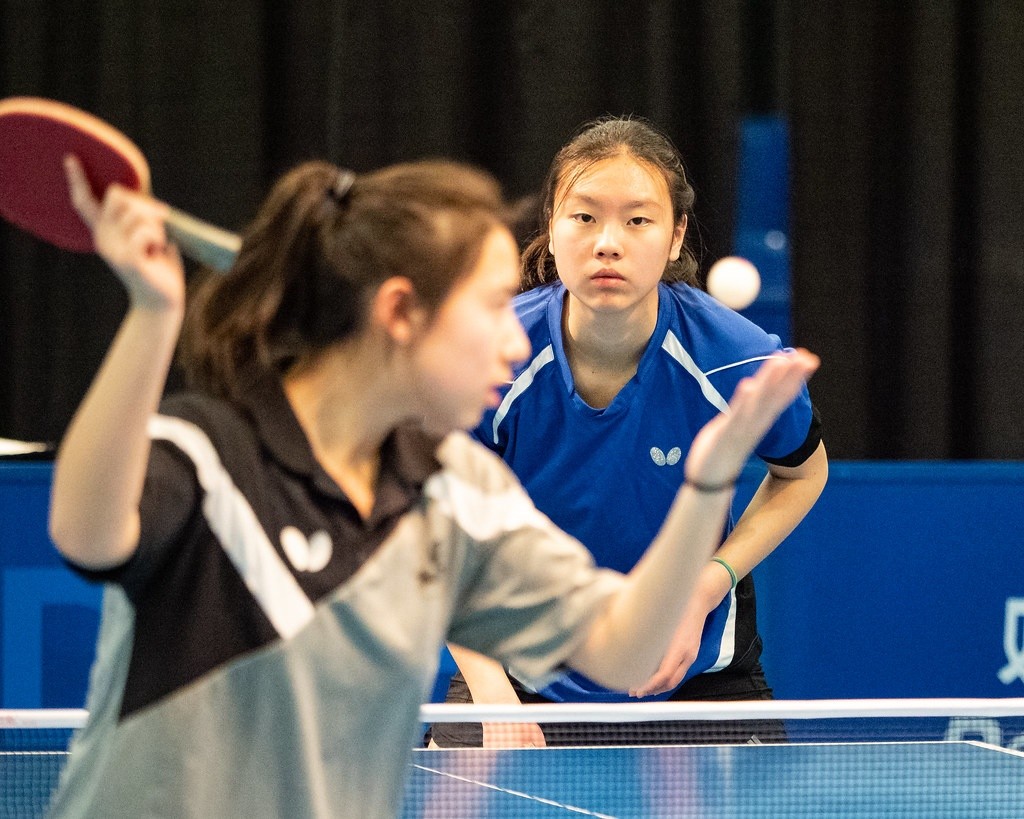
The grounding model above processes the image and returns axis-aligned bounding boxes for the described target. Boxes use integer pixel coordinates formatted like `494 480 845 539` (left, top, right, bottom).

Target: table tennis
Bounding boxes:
707 253 763 304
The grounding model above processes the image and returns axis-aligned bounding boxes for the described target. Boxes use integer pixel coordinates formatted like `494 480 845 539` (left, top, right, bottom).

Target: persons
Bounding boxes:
423 115 829 750
43 154 818 819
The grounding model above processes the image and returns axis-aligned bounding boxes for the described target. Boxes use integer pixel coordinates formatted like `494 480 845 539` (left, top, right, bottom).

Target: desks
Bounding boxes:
0 740 1024 818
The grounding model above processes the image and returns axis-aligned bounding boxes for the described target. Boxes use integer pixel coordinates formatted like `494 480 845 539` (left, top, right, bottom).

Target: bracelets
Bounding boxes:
680 469 741 498
710 555 736 591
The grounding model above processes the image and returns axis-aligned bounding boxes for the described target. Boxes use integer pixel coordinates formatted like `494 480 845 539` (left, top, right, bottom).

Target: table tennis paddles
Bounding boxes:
0 96 245 274
